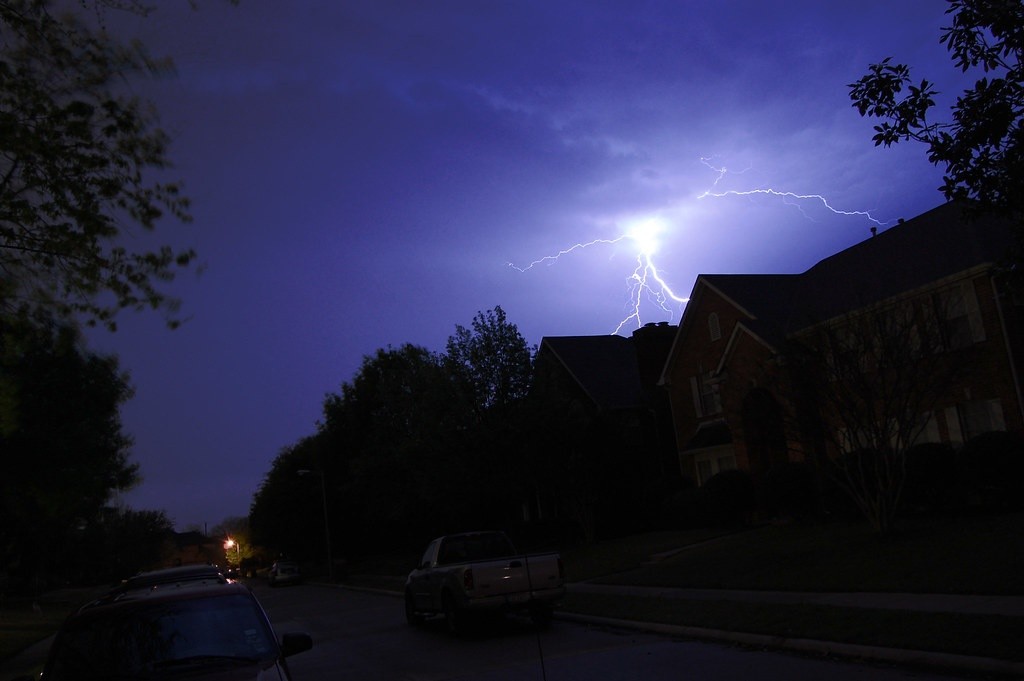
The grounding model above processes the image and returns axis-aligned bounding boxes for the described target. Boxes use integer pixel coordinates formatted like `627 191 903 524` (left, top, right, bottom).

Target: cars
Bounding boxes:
11 573 312 681
96 545 307 605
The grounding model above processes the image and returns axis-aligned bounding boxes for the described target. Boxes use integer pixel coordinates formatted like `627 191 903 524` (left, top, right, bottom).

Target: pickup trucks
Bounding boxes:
404 531 570 638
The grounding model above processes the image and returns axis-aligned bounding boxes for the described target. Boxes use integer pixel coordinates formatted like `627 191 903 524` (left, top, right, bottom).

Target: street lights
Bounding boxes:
297 470 333 582
